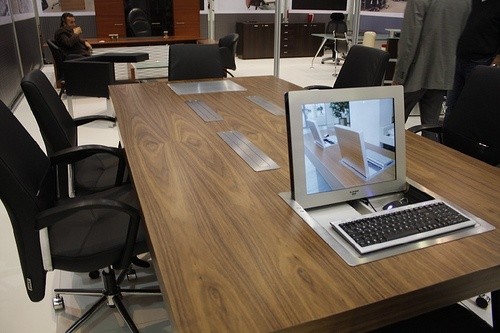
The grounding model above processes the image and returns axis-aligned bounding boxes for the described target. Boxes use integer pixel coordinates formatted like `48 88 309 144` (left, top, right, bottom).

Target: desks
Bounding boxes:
310 33 413 72
304 128 396 189
94 50 149 79
107 74 500 333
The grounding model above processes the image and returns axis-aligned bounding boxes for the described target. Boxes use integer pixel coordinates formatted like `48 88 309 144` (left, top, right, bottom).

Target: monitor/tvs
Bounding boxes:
285 84 407 209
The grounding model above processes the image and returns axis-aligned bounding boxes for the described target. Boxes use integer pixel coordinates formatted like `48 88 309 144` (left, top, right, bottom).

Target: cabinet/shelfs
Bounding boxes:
235 21 326 60
94 0 199 37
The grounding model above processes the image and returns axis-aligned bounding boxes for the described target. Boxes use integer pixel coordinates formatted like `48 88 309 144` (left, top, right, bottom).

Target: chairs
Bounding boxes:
408 66 500 167
167 46 237 79
321 12 349 64
47 39 89 88
128 8 154 37
219 34 240 78
304 45 391 91
0 69 153 333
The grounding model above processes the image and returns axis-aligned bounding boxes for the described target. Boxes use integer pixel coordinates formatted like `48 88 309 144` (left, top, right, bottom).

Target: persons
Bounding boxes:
55 12 93 56
392 0 471 140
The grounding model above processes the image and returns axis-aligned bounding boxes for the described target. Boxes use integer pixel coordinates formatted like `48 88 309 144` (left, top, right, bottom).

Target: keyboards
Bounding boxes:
330 199 476 254
